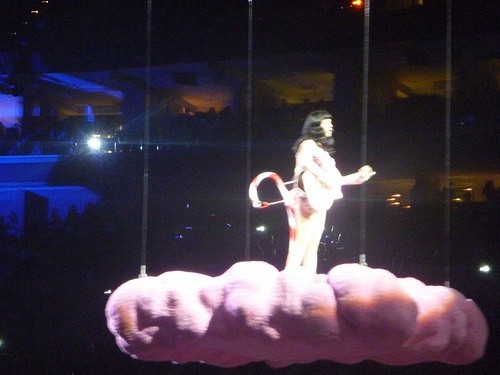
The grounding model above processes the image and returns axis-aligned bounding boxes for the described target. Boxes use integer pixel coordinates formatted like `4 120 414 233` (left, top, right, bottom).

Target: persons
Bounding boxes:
279 108 376 273
